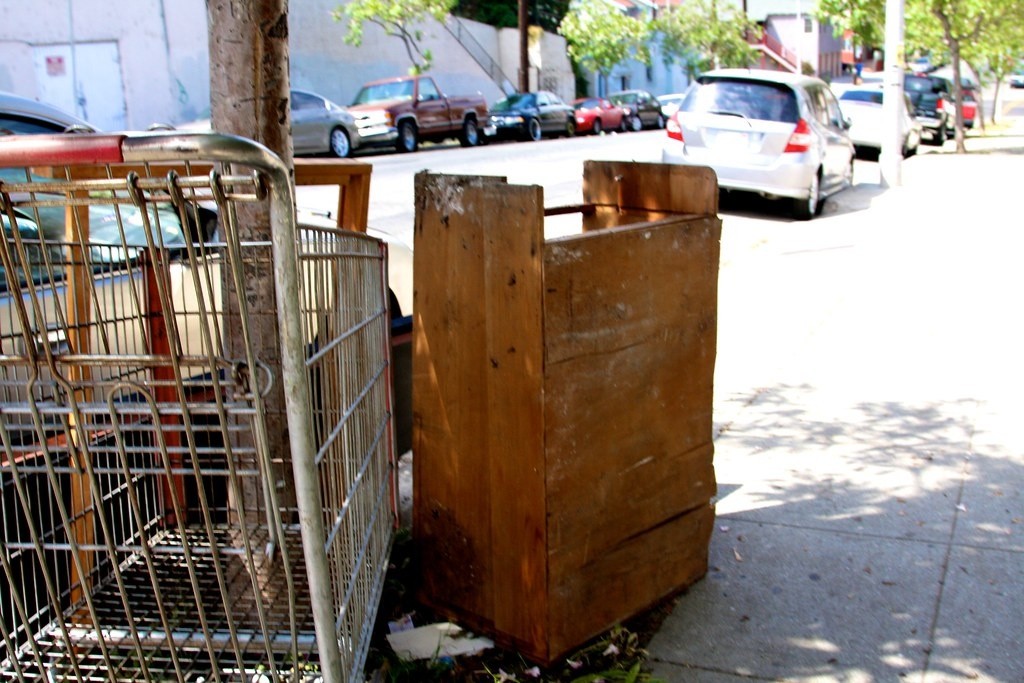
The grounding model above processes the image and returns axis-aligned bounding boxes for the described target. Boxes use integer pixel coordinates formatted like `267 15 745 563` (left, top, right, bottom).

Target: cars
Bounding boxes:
476 91 574 145
661 68 857 221
839 73 977 156
1 92 413 436
569 97 628 136
179 89 361 161
608 90 687 132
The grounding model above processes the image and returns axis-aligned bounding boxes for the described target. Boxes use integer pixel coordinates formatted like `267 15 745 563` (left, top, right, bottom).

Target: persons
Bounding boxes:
854 58 865 84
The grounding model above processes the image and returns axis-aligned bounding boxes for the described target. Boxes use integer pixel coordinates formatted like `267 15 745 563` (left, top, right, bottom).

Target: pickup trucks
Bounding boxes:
343 74 490 152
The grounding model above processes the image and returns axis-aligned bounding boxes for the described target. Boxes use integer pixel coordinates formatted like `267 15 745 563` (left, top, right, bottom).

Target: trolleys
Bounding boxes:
0 125 402 683
